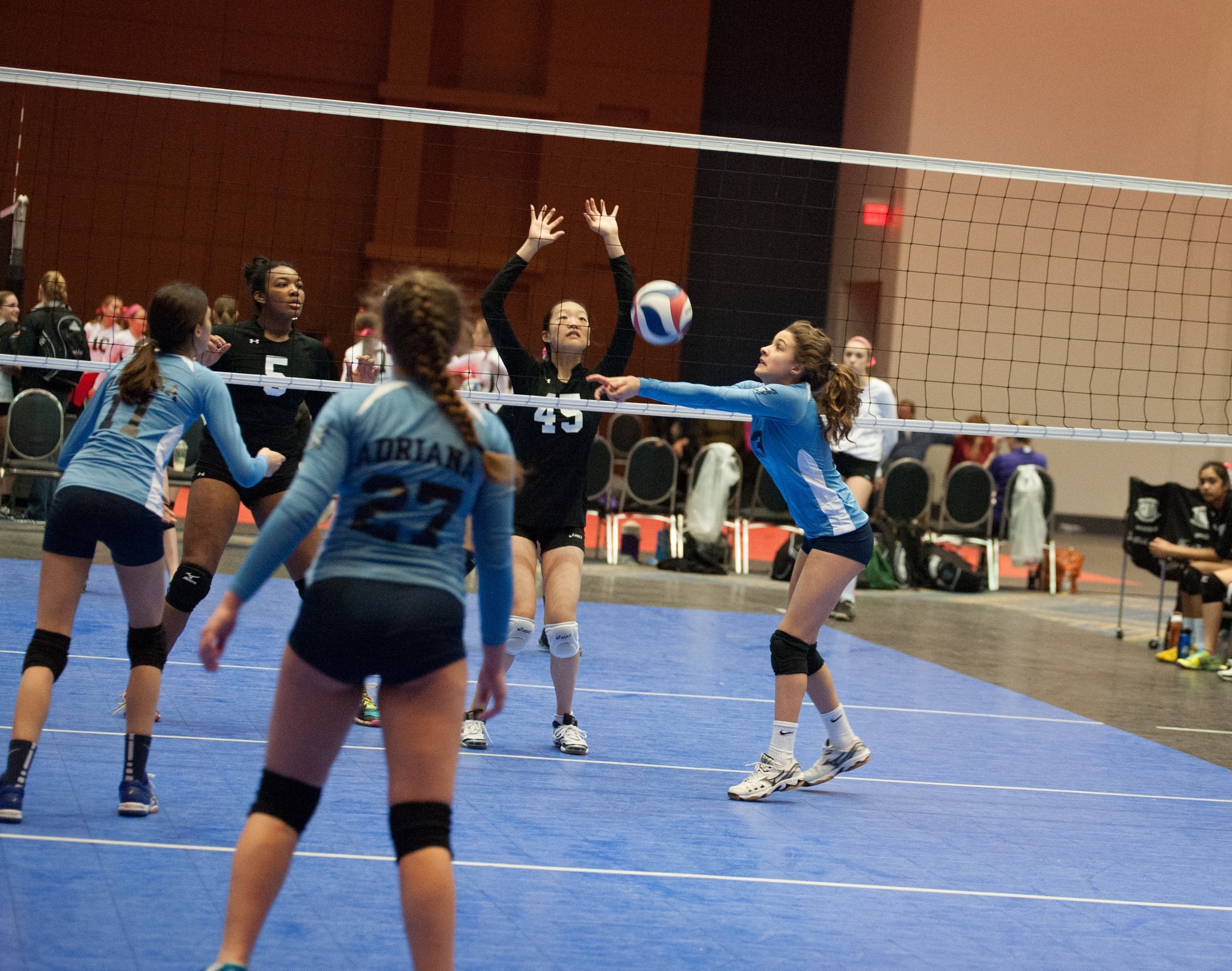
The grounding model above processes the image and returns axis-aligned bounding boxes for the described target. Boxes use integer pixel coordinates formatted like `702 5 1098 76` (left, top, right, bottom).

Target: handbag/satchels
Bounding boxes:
917 541 980 592
1038 547 1084 594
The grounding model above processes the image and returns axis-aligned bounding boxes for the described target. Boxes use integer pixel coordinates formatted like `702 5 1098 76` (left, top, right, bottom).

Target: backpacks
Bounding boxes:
770 533 808 582
656 522 731 576
860 536 906 590
30 301 91 390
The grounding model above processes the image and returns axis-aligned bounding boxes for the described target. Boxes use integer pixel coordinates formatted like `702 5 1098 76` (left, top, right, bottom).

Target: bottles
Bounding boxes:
1177 627 1192 660
1166 610 1183 650
621 521 639 561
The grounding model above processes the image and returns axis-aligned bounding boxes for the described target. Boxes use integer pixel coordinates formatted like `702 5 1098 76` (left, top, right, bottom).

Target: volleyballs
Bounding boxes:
629 280 693 347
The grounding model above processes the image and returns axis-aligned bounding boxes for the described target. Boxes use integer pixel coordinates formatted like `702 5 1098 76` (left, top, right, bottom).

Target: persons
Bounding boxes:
587 320 870 801
0 257 475 729
199 272 516 970
651 335 1048 621
0 282 285 823
460 199 637 752
1148 461 1232 681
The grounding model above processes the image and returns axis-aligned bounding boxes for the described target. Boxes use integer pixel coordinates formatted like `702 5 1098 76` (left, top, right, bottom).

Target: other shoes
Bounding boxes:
1215 666 1232 681
206 962 247 971
829 598 856 621
0 505 46 525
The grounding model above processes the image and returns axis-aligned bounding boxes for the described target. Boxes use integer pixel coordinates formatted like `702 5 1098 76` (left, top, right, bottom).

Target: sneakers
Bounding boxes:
108 689 161 722
801 737 871 787
118 779 158 816
727 753 805 801
552 713 589 755
1176 648 1222 671
352 683 382 727
0 774 25 823
1156 646 1178 663
459 709 494 749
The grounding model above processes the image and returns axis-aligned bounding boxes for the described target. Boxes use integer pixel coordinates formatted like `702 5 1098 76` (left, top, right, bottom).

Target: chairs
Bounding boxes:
1115 475 1202 649
165 413 206 485
581 414 1058 595
0 389 64 521
